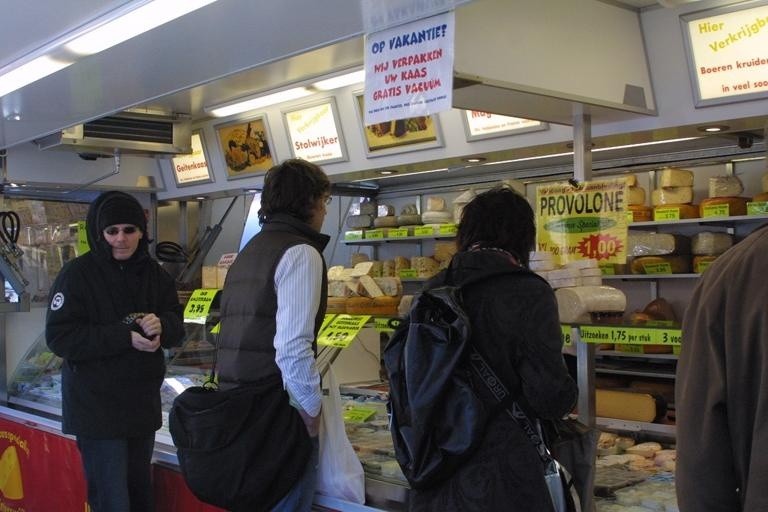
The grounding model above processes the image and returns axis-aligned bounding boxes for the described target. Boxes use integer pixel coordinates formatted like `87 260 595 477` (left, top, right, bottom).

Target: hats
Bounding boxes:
99 196 146 233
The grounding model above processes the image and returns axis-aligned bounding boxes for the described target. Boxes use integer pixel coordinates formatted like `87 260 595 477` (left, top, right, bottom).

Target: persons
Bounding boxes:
44 190 188 512
216 157 334 512
406 185 584 510
672 222 767 512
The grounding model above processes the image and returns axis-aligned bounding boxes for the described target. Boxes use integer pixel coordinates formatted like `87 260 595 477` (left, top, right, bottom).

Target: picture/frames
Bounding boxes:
678 0 768 109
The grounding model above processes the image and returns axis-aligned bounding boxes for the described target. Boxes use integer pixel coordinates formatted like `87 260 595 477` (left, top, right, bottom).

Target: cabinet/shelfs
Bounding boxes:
313 139 768 440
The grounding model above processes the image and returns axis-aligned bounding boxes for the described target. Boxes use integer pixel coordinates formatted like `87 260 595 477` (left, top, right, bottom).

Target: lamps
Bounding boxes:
203 64 365 118
0 0 218 97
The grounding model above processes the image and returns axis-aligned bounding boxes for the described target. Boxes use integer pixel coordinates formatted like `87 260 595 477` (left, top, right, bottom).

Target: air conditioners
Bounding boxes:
40 108 193 156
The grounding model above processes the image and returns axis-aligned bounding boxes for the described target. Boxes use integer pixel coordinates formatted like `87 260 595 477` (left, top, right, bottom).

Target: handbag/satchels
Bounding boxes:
169 368 322 512
546 457 580 511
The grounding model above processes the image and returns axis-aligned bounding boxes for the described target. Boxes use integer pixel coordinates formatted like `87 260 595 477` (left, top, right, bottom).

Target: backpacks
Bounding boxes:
383 269 522 491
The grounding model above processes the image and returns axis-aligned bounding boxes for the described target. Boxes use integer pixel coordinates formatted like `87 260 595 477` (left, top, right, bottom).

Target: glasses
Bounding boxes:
319 195 333 205
106 226 135 234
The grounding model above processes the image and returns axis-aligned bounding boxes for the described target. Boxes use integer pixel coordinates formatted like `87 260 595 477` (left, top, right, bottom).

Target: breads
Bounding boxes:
345 200 453 234
326 265 416 319
625 229 743 276
595 431 679 512
618 167 768 219
350 251 437 278
